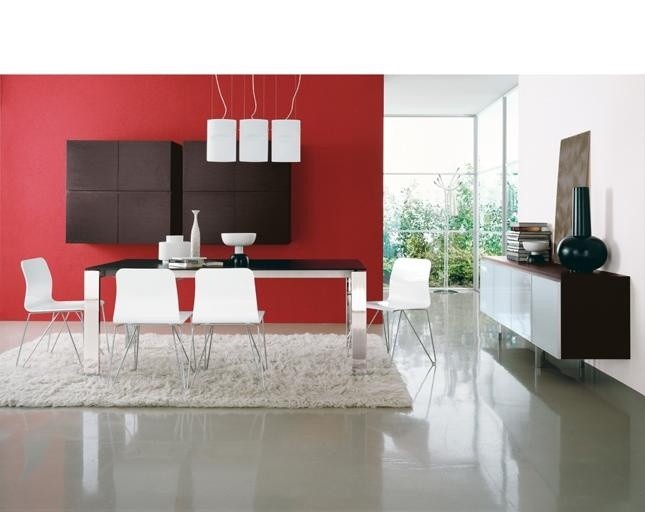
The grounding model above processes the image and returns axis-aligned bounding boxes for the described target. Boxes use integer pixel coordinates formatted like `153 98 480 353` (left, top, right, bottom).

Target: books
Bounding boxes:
168 263 203 269
168 258 204 264
505 221 552 263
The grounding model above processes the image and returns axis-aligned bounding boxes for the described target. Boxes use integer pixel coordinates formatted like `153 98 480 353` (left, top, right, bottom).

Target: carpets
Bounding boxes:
0 332 414 409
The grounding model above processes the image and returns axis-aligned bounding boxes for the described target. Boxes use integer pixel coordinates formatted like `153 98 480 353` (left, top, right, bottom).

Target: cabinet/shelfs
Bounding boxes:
65 140 291 245
480 258 629 376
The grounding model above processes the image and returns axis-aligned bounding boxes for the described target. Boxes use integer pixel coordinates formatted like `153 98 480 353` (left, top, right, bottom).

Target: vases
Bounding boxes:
190 209 201 257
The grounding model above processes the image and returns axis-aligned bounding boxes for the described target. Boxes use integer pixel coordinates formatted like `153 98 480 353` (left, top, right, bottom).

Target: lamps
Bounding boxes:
206 74 302 164
